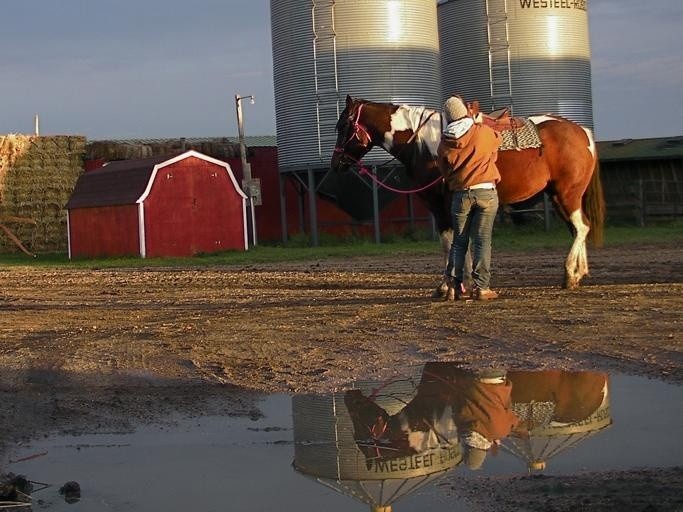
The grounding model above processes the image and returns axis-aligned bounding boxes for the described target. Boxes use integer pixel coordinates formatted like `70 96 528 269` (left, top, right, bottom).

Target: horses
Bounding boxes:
330 94 607 299
343 362 610 471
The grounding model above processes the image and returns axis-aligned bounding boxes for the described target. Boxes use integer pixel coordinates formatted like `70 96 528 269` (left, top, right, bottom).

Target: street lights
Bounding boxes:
233 91 258 247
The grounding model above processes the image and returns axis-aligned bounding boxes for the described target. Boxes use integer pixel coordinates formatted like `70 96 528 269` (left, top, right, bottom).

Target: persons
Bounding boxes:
447 366 519 469
431 95 501 301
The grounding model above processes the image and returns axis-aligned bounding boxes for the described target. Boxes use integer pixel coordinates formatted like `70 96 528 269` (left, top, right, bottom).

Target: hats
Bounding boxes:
463 446 486 471
444 97 468 123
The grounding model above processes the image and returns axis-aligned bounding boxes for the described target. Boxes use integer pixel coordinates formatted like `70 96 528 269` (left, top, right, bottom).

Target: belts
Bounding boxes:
463 182 494 190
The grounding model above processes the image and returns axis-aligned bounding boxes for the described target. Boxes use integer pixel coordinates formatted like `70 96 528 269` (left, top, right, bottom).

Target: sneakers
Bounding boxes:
446 288 461 301
476 288 498 301
